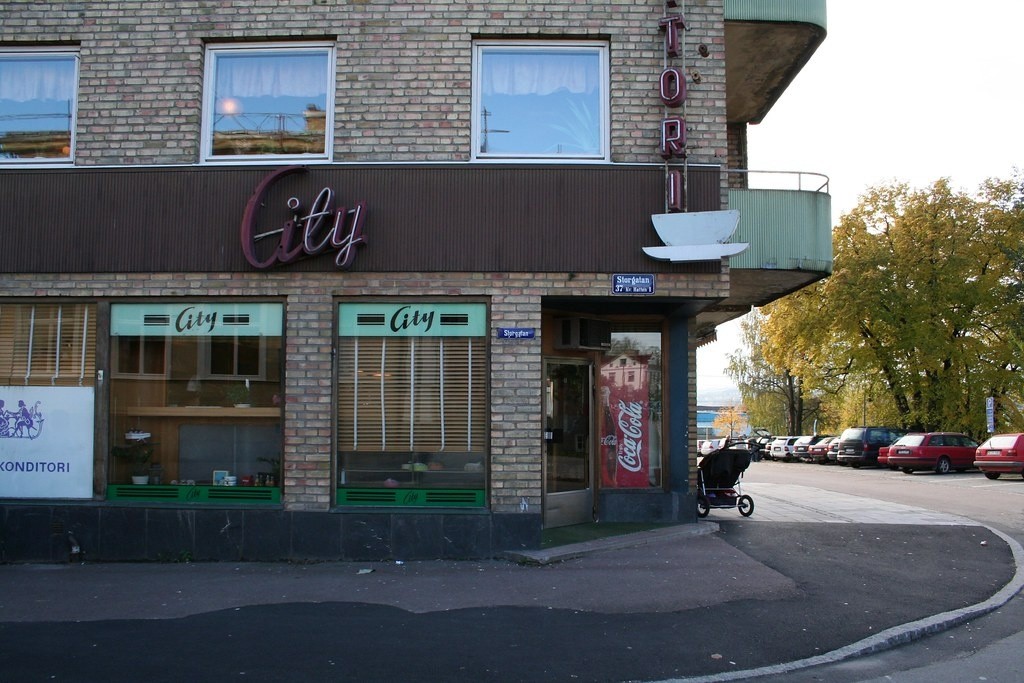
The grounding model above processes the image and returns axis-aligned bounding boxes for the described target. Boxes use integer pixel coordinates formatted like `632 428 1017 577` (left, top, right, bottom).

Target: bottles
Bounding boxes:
254 475 259 486
259 475 264 487
265 474 271 487
270 476 274 487
600 386 618 488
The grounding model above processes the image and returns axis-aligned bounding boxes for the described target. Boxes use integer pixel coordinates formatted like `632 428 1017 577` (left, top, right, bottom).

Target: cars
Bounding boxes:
973 433 1024 480
877 437 905 470
696 433 848 467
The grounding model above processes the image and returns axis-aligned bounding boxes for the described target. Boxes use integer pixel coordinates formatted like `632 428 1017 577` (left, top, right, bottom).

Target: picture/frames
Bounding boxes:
212 470 228 486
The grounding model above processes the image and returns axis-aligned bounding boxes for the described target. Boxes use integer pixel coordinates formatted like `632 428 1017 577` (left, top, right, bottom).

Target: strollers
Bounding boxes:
697 441 762 517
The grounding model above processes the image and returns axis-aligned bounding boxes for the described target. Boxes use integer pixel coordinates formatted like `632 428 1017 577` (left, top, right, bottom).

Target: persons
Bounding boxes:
735 434 745 448
725 434 733 448
750 436 760 462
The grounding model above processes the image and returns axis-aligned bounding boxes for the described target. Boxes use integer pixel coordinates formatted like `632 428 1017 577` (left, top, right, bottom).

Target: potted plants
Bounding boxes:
111 442 160 485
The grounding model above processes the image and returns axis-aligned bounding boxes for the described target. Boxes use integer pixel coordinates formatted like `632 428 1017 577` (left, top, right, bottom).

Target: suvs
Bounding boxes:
887 432 982 476
837 426 907 469
